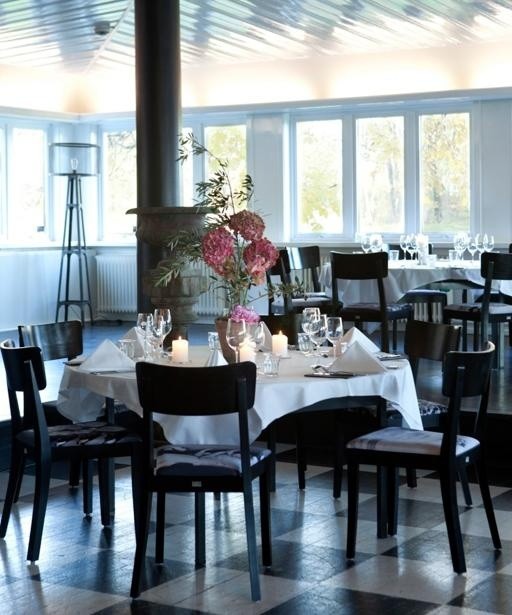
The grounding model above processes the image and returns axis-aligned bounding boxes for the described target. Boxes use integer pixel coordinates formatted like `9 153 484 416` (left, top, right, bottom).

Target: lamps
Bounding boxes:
46 140 105 326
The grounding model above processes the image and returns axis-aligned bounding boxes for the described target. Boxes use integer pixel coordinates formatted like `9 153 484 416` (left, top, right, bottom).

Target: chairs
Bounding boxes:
0 235 512 606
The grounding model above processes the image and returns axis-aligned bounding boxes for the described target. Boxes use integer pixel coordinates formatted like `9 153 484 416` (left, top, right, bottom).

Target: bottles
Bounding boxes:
204 333 225 366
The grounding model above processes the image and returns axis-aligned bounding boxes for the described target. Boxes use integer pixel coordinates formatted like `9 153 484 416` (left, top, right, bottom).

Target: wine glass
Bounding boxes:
297 308 343 368
227 317 264 375
356 230 496 267
132 307 172 365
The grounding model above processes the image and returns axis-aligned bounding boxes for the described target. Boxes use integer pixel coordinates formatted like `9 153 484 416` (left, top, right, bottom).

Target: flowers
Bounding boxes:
136 130 304 323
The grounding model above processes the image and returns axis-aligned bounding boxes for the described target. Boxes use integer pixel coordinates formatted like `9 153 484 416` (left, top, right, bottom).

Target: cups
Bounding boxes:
117 340 134 358
263 353 284 378
